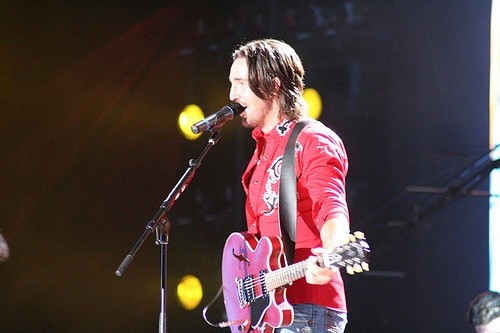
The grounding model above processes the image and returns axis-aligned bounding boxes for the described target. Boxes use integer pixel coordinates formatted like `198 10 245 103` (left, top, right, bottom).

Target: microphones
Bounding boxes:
191 102 243 134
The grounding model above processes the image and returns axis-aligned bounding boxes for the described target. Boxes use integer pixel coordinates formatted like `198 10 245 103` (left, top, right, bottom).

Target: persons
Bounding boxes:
211 39 371 333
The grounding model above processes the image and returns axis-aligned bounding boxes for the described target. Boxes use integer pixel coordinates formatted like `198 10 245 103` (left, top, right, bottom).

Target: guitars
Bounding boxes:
221 231 372 333
